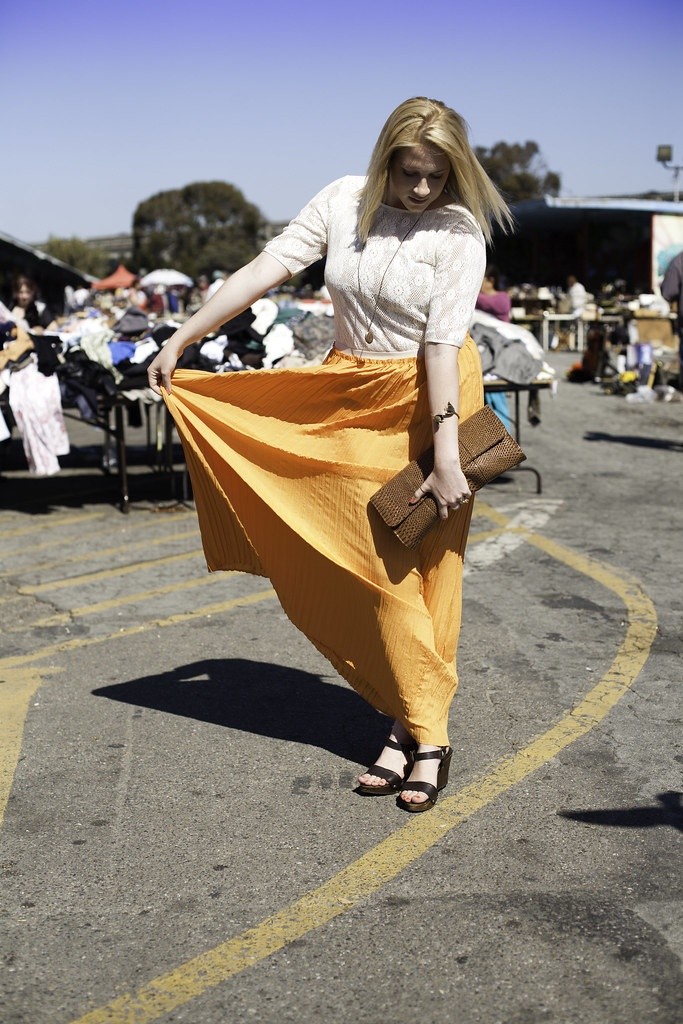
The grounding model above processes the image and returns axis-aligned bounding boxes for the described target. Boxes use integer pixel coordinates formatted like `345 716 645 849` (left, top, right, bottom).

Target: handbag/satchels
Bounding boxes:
370 406 528 550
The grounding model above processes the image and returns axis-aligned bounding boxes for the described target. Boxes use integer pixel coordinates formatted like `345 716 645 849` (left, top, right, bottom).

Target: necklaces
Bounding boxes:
357 203 432 344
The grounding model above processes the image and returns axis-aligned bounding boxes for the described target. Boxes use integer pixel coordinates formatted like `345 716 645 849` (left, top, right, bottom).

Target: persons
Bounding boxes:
0 275 69 476
198 272 278 370
568 276 587 310
147 97 514 812
605 326 630 372
322 285 335 318
660 251 683 332
64 283 191 319
475 265 511 434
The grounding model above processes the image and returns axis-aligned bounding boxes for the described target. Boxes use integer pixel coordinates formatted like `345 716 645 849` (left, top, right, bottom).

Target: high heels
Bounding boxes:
358 740 421 794
399 746 452 812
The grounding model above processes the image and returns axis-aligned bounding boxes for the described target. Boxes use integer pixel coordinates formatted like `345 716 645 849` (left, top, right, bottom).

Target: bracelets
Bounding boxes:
431 402 460 425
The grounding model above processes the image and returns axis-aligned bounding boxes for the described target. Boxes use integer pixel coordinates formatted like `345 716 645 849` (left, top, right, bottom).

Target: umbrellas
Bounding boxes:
140 268 192 287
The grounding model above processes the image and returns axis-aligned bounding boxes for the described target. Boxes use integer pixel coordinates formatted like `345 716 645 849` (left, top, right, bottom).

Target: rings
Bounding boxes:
460 499 469 505
452 505 459 510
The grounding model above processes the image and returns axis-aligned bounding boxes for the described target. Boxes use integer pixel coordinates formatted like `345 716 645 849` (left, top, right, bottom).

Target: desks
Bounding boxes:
482 378 553 493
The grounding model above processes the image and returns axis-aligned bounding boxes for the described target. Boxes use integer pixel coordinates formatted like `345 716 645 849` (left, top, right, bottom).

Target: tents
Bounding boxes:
92 265 135 288
